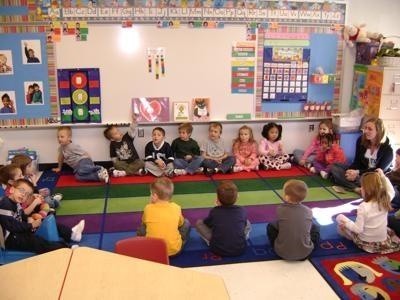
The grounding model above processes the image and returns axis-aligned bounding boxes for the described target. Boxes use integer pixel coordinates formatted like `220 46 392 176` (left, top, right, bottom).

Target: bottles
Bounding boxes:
303 101 332 117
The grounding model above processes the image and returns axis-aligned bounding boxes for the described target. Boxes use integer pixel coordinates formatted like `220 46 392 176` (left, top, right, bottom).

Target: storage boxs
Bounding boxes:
331 112 362 128
355 41 394 65
376 56 399 68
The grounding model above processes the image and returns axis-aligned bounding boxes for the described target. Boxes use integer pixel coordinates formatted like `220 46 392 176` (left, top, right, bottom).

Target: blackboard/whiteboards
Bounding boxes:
0 0 349 129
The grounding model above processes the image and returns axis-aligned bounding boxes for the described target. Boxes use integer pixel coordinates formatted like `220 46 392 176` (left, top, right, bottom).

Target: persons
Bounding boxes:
326 118 393 196
0 93 16 114
142 126 176 178
1 165 56 221
1 155 63 209
258 122 292 172
52 126 111 183
202 122 236 174
290 119 334 172
231 125 262 174
46 19 81 44
135 176 192 258
1 179 71 252
308 133 344 179
382 146 400 239
31 83 43 105
102 112 148 178
336 174 396 255
169 120 205 176
194 181 252 257
156 18 209 30
24 42 41 64
0 54 12 73
25 83 34 104
264 178 322 260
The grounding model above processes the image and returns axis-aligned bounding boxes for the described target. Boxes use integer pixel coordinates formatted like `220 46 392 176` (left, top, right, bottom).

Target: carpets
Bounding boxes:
0 163 400 300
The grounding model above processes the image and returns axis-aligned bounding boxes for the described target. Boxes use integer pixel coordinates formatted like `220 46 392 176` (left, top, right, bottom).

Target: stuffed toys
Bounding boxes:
343 24 382 48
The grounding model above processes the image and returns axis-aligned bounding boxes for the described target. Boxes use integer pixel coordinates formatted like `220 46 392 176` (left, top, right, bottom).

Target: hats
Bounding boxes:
262 123 282 142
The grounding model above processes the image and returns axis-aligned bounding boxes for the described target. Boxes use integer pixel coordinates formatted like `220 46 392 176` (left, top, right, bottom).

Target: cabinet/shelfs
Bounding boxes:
351 63 399 149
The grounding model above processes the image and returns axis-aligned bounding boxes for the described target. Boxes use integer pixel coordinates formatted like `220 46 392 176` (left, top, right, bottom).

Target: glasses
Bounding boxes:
17 188 31 198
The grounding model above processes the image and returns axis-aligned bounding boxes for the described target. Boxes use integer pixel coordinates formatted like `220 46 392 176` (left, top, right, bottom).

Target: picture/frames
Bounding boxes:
173 101 190 123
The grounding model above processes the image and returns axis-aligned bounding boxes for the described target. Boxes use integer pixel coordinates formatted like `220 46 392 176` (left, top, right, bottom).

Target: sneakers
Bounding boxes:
98 169 109 183
207 168 216 176
52 193 63 201
165 169 173 177
279 162 291 170
145 169 148 174
71 220 85 241
320 171 328 180
310 167 315 173
253 166 258 170
305 163 311 169
174 169 186 176
233 166 243 172
196 167 203 173
113 170 126 178
136 169 142 176
